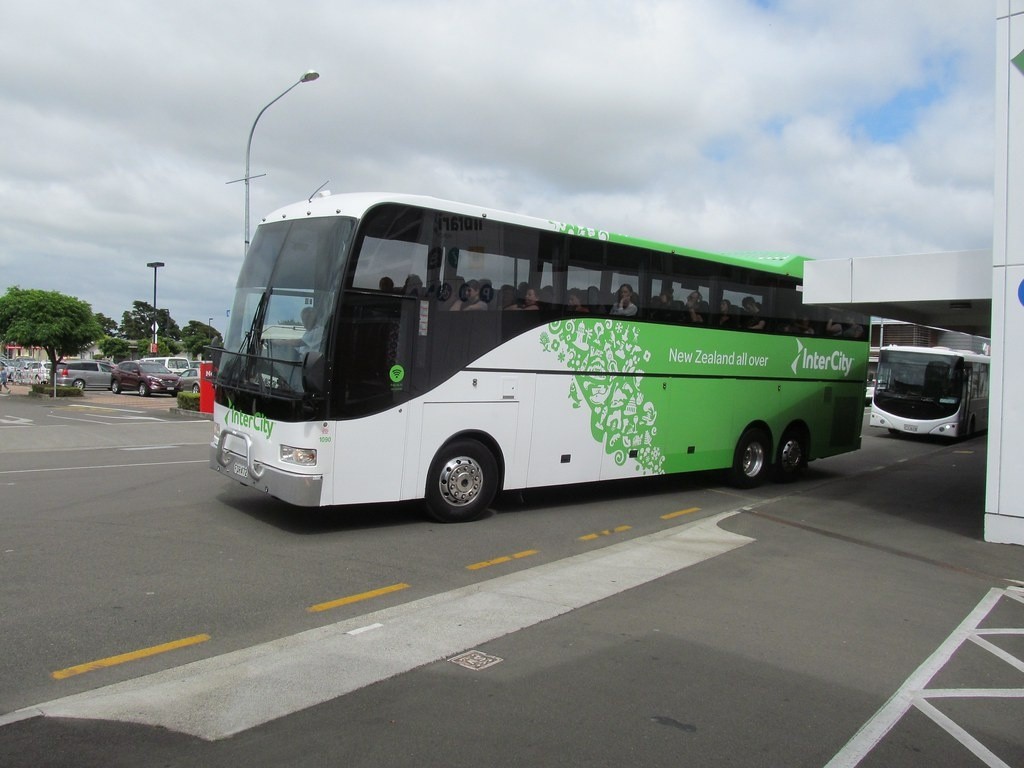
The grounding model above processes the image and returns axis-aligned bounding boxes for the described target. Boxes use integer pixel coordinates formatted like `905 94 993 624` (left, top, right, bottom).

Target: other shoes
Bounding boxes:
7 389 11 394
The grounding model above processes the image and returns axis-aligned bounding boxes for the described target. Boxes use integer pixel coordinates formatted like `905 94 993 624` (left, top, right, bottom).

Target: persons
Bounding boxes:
0 367 11 394
378 276 865 340
260 307 324 361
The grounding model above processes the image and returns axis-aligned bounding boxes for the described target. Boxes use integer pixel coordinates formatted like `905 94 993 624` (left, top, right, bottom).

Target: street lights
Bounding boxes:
244 70 320 257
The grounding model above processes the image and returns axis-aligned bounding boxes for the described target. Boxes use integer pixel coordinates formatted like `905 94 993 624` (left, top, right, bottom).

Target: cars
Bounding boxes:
190 361 213 369
0 352 70 384
177 368 200 394
110 360 184 398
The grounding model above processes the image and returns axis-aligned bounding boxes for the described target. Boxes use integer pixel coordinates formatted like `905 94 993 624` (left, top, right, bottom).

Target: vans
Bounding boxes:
141 356 190 377
51 359 119 391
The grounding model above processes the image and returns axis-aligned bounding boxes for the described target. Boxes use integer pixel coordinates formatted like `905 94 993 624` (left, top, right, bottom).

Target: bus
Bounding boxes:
869 345 990 440
208 191 875 523
